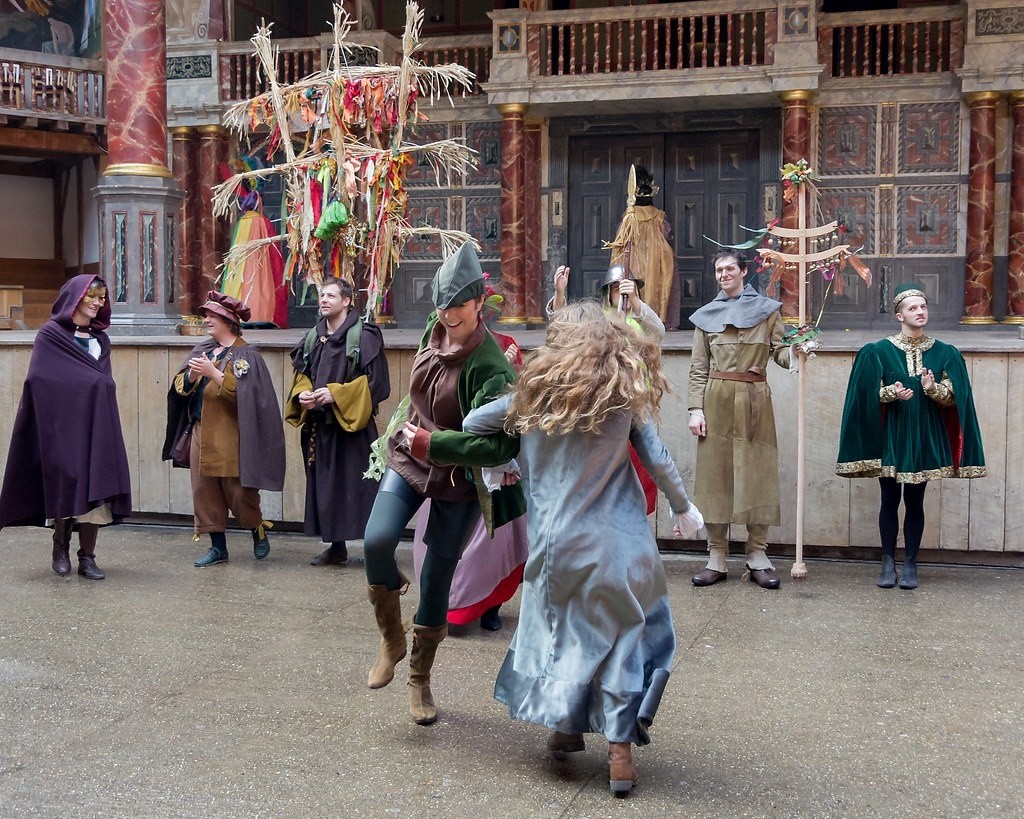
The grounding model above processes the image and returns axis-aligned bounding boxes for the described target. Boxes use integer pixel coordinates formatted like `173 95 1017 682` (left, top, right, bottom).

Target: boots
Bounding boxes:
51 518 76 574
604 742 637 791
76 522 105 579
366 558 412 687
545 729 587 754
406 613 449 725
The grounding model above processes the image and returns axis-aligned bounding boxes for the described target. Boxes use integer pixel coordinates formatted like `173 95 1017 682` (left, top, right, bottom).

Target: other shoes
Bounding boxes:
481 603 503 631
750 567 780 589
310 541 348 565
691 568 728 586
876 555 897 587
898 558 918 588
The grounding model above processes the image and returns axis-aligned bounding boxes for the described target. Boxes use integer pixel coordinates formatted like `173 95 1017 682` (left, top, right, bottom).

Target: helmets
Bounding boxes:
599 264 646 294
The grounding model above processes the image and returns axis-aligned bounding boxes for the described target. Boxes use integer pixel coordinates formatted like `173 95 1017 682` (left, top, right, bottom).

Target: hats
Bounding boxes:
198 290 252 326
893 281 928 309
431 241 485 310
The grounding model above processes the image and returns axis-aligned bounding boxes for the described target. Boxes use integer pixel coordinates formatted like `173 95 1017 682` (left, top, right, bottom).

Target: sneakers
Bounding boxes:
194 546 229 566
250 529 270 559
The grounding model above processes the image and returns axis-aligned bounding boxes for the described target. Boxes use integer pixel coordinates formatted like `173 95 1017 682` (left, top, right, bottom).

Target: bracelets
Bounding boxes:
690 413 704 418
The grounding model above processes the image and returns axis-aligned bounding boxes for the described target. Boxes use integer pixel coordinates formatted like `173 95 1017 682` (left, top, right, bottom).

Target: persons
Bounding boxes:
545 264 664 516
462 302 705 799
685 250 821 588
0 275 132 580
162 290 286 566
286 276 398 566
413 331 527 633
835 284 987 589
364 240 522 724
221 155 290 329
610 165 681 330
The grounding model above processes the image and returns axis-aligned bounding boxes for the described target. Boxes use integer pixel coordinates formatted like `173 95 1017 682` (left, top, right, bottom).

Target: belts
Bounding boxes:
709 367 767 383
